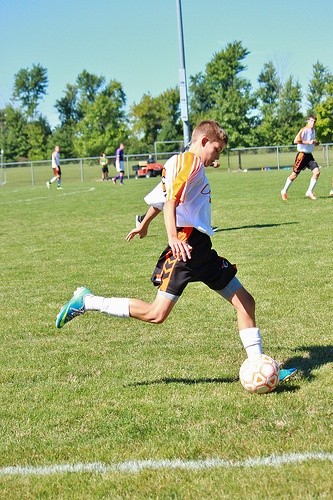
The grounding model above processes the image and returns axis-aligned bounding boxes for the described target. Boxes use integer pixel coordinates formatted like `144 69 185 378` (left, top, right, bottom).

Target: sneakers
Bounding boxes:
278 368 296 382
55 287 92 329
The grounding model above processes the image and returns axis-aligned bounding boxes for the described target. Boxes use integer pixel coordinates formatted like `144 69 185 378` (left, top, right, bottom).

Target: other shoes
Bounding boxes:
45 182 50 189
305 192 316 199
281 190 287 200
111 178 116 184
134 215 140 228
56 187 61 189
210 226 217 232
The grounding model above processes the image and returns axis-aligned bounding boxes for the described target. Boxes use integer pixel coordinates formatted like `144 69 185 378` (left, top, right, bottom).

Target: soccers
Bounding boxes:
238 354 280 394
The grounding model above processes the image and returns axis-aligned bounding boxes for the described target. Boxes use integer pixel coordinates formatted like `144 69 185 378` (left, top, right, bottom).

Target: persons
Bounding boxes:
280 115 321 200
56 121 297 385
46 143 220 232
